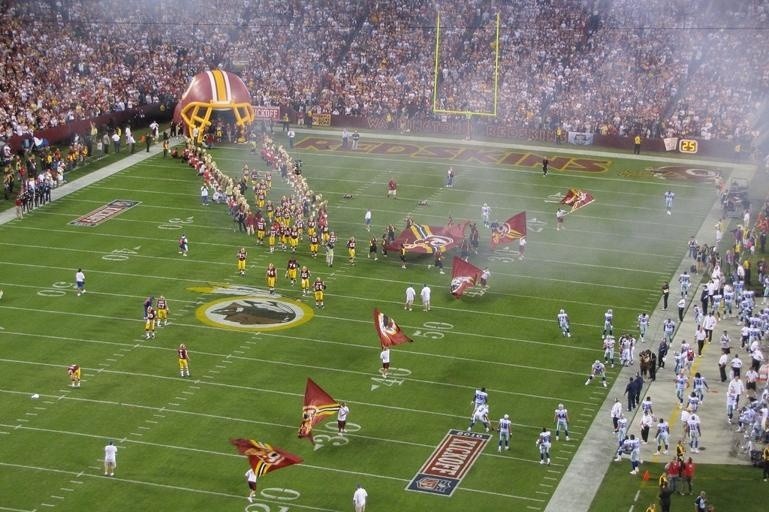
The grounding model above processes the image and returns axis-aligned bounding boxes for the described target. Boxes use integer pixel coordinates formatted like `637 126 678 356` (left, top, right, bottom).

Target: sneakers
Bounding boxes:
382 373 387 378
604 360 633 369
654 450 668 455
612 429 618 434
640 335 645 343
486 428 489 432
602 334 605 340
567 333 571 337
630 467 639 475
248 493 255 503
585 380 591 385
641 439 648 444
105 472 114 477
555 436 559 440
602 383 607 387
718 314 742 325
562 332 566 336
405 307 430 312
497 446 509 452
680 491 693 496
612 335 614 338
728 417 745 432
467 427 471 431
614 455 621 462
338 429 347 436
566 436 571 441
540 459 550 464
691 448 700 452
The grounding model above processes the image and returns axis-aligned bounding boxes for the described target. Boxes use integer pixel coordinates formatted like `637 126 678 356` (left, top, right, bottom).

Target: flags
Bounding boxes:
228 438 305 478
298 378 342 446
373 306 416 350
449 257 484 300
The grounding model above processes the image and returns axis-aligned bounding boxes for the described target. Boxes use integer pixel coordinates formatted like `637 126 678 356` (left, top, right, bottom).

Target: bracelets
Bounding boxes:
510 433 513 436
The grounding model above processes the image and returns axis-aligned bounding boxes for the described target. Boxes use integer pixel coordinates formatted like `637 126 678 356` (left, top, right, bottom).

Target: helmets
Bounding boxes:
504 415 509 419
606 334 611 339
560 309 564 313
558 404 564 409
595 360 600 364
608 308 613 314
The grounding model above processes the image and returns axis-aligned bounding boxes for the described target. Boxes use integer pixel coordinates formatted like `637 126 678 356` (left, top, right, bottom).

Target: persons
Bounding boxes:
467 404 491 434
470 386 489 423
1 3 768 278
245 466 258 504
353 483 368 512
604 335 616 370
380 346 391 380
602 309 615 340
145 307 156 341
156 295 173 328
336 402 351 434
481 267 491 296
553 403 571 442
103 440 118 478
312 278 327 311
612 278 769 512
497 414 513 454
585 360 609 389
536 426 553 465
420 283 433 313
300 266 313 297
68 365 81 388
143 296 157 320
75 268 87 298
265 263 278 297
557 307 573 339
285 256 300 289
403 284 418 313
177 344 192 377
